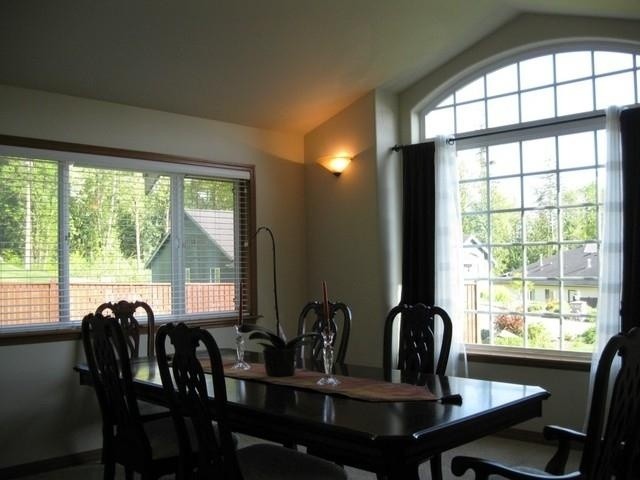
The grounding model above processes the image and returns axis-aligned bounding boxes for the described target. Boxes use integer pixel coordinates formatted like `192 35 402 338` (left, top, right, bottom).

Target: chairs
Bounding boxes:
82 313 239 480
450 325 640 480
383 302 454 479
94 300 157 465
156 323 348 479
296 300 353 374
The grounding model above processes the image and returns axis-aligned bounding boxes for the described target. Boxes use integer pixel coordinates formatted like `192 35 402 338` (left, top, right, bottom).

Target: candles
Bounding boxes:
321 279 332 342
238 282 244 325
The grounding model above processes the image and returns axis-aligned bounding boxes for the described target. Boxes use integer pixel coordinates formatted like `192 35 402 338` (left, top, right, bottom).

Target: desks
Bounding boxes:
72 350 550 480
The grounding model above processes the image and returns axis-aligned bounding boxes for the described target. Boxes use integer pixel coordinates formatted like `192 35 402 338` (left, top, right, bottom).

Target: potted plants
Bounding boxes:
237 324 327 377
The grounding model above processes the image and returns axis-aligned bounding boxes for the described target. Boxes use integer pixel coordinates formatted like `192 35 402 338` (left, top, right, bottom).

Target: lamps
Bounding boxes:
313 153 353 176
